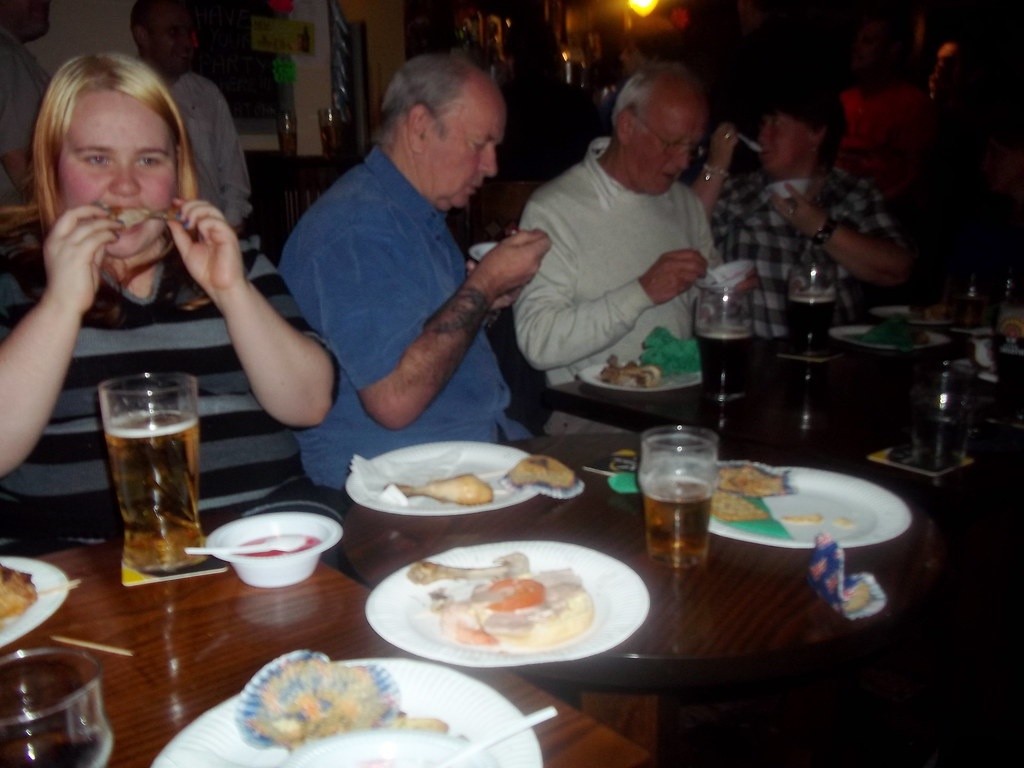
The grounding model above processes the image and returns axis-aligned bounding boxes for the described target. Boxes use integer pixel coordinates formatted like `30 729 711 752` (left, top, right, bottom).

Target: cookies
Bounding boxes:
712 490 764 522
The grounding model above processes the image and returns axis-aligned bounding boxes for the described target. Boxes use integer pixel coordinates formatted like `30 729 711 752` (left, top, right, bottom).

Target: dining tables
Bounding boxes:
0 315 1024 768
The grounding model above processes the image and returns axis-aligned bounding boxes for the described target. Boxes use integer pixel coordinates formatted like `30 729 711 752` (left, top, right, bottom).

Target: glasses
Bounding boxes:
631 109 706 162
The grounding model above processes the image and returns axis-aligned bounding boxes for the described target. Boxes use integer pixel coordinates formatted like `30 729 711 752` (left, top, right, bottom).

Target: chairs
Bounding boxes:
469 181 543 243
282 165 348 239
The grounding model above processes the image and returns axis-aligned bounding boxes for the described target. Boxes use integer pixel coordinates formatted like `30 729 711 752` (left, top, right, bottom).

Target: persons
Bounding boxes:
0 51 335 553
724 0 1024 288
282 56 551 492
691 92 910 336
0 0 51 206
513 64 758 434
129 0 251 228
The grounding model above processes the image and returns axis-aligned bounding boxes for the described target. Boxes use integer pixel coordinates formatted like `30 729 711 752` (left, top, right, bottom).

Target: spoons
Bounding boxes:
736 133 762 153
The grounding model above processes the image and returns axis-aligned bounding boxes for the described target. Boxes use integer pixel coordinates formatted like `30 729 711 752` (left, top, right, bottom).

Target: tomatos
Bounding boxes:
486 578 545 610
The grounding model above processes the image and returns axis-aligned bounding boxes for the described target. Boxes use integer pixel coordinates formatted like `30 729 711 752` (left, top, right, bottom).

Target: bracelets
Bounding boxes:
703 164 728 181
812 219 838 246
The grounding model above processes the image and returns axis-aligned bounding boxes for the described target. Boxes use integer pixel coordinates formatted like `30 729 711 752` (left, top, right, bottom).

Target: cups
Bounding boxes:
694 288 756 403
1 648 115 768
637 425 717 566
317 108 343 160
275 113 298 156
97 370 201 569
909 360 973 472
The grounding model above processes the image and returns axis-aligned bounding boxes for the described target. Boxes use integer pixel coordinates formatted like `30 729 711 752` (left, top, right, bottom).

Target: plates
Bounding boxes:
828 324 950 351
344 439 538 517
708 466 913 549
576 361 703 391
363 540 650 666
0 556 69 650
869 301 949 324
150 658 543 768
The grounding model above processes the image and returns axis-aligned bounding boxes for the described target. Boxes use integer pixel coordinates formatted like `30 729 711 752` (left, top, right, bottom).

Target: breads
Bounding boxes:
466 574 592 648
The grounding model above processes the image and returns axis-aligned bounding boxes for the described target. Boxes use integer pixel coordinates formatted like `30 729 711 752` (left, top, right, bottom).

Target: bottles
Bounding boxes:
786 238 837 350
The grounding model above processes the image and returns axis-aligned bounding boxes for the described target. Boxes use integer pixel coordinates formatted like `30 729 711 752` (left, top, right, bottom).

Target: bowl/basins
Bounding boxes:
283 729 499 768
696 259 752 294
205 510 343 589
468 242 498 265
765 177 810 200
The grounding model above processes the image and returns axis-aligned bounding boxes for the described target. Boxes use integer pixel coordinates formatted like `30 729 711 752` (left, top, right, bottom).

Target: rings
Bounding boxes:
788 208 795 214
724 132 730 138
